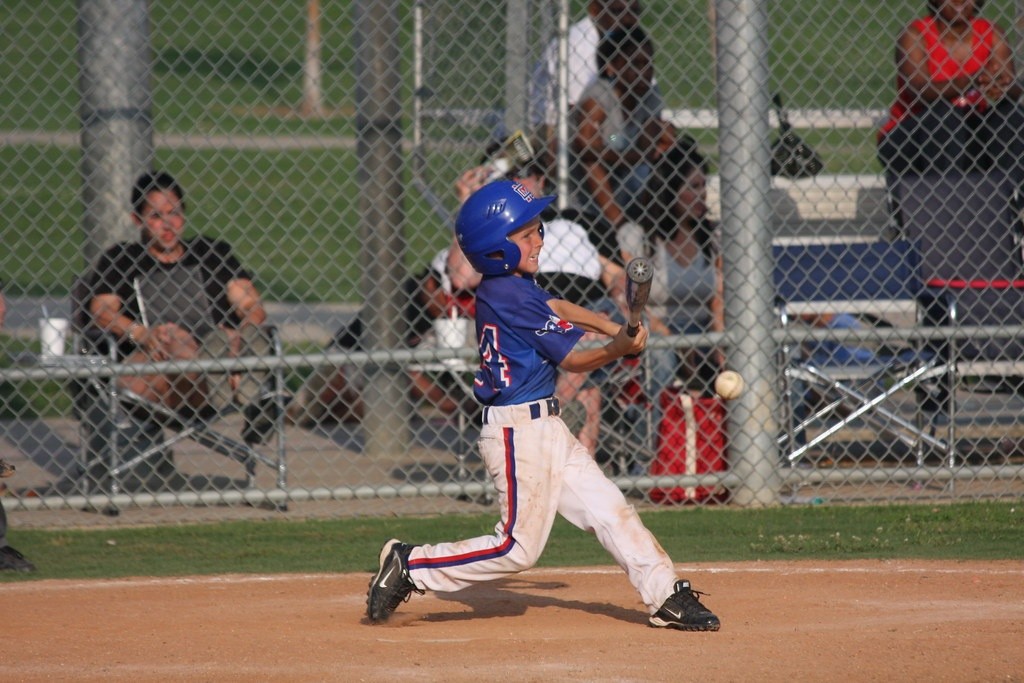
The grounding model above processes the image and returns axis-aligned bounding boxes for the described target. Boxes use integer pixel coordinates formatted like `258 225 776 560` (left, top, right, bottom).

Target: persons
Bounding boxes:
366 180 720 632
284 0 721 456
89 171 266 409
876 0 1016 235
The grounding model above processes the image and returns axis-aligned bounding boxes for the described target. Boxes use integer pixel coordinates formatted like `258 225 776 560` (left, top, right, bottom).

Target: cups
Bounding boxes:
38 318 68 357
434 318 467 365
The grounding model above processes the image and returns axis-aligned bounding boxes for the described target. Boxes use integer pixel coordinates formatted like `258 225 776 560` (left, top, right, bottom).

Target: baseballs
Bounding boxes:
715 369 745 399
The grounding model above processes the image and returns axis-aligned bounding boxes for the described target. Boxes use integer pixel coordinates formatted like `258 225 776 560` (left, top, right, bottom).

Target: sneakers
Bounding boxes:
649 578 721 632
364 538 426 625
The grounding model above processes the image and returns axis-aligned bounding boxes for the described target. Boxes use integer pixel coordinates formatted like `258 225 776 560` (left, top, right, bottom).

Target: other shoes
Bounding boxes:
197 328 233 411
236 324 267 406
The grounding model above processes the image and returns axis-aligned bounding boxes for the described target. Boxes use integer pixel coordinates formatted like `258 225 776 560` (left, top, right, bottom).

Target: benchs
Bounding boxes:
698 174 906 247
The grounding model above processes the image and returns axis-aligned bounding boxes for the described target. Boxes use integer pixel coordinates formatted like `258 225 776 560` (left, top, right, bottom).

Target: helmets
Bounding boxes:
455 180 557 275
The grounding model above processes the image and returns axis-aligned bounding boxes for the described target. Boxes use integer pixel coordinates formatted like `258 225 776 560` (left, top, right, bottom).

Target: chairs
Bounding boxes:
70 276 286 515
430 247 654 505
770 294 929 468
885 169 1024 467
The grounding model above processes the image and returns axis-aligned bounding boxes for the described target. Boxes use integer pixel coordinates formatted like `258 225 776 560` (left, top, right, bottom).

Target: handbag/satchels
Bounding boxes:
768 92 823 179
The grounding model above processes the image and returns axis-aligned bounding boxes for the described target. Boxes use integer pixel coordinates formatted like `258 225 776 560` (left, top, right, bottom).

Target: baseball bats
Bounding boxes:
623 255 656 359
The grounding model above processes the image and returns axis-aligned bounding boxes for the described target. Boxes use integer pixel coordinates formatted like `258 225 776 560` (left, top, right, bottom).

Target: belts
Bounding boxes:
484 396 560 424
